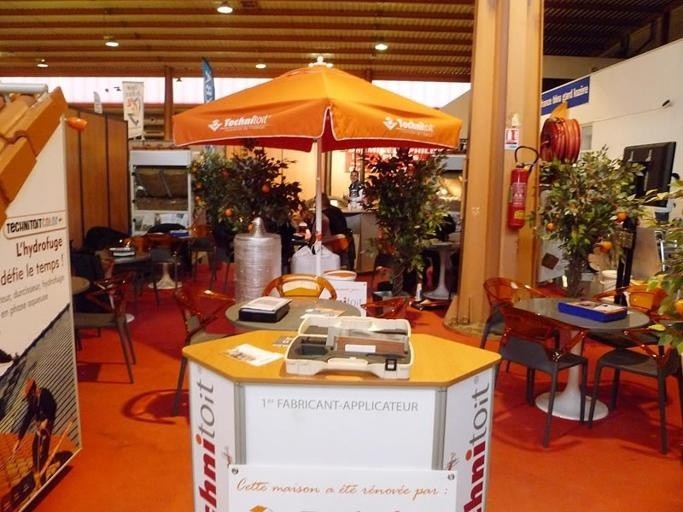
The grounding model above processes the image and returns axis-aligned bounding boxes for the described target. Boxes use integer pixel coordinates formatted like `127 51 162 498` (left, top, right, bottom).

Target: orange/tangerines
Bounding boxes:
617 212 627 222
546 222 556 232
224 208 233 217
194 195 202 207
195 182 203 189
262 183 271 193
248 224 253 230
600 241 612 253
223 168 230 177
673 300 682 315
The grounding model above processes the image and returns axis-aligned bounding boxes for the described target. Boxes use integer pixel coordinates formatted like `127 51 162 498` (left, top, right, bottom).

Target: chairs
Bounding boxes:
590 284 667 397
67 222 227 417
591 328 682 453
373 225 404 293
496 300 590 449
261 274 338 300
361 293 417 320
626 310 682 432
480 273 579 359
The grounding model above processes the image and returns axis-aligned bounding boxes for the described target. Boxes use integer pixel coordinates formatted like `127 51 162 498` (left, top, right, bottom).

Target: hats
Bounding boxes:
22 379 35 402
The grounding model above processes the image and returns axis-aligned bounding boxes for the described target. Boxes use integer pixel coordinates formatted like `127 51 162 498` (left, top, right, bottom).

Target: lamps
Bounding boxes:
216 0 234 14
105 38 120 49
36 58 48 68
63 116 90 133
255 58 266 70
374 36 389 51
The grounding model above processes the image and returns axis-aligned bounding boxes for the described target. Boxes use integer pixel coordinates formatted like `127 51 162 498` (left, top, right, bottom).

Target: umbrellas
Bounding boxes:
168 58 466 272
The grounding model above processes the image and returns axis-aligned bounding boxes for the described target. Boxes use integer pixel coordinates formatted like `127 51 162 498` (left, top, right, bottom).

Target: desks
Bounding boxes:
224 296 361 332
417 235 459 300
515 299 650 420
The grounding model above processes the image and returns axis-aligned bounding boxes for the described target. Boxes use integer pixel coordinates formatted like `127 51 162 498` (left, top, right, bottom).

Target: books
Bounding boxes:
106 243 131 252
238 296 293 325
109 250 135 258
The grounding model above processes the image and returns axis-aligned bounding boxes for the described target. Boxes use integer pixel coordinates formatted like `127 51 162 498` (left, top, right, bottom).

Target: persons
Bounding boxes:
211 204 314 266
420 193 455 289
309 191 357 273
10 377 57 487
340 169 365 207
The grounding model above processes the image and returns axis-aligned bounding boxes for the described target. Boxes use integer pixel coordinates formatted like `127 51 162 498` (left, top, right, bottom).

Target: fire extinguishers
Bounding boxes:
507 145 539 229
